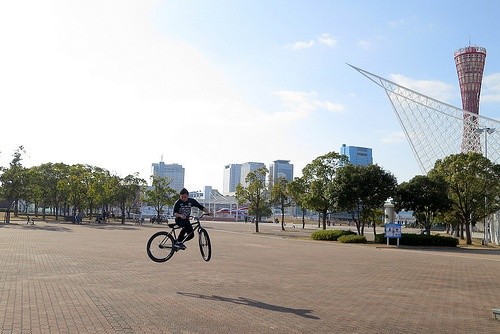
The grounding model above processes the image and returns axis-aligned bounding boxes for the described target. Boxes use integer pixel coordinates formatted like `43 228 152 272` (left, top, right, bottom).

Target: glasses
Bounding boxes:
181 196 187 199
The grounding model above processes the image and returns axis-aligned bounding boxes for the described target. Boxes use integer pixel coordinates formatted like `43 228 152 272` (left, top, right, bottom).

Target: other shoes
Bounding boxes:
174 242 187 249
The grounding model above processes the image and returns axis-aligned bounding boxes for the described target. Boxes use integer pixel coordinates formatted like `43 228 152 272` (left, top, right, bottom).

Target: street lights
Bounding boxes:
474 125 496 247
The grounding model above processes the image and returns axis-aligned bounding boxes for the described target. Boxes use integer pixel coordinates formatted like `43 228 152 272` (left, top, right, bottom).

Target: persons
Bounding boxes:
141 215 144 225
244 214 247 223
172 188 208 249
76 212 81 225
149 214 167 224
96 210 110 222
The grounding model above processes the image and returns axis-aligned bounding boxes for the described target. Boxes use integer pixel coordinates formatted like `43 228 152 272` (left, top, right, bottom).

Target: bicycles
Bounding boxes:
147 213 212 263
